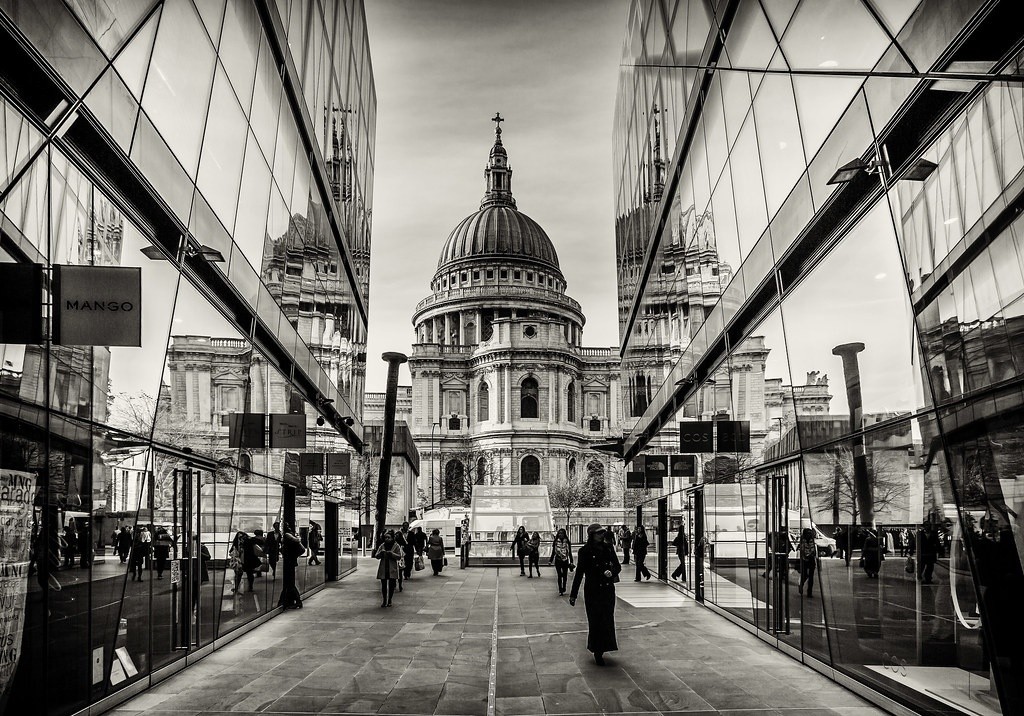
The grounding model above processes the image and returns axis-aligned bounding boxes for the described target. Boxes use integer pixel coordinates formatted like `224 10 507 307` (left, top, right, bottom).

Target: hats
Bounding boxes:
587 523 606 536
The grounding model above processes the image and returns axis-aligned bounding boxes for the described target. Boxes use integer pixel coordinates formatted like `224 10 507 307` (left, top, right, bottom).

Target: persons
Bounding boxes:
671 526 689 581
283 523 305 610
548 529 573 595
112 525 174 581
570 524 621 665
31 518 89 569
375 521 445 607
605 526 616 545
619 525 651 582
300 525 321 565
229 523 281 592
510 526 541 578
762 505 1018 636
201 545 211 585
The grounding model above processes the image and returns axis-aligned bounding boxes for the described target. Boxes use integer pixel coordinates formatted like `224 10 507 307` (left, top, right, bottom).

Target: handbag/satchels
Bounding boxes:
672 534 683 546
258 556 269 572
645 539 648 545
444 557 447 566
905 556 915 574
397 547 405 569
858 557 865 568
609 564 620 583
229 546 242 571
415 556 425 571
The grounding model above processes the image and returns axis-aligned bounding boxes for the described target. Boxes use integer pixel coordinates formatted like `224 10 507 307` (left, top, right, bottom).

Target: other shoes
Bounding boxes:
399 586 403 591
528 575 532 578
308 561 312 566
671 574 676 581
593 655 604 666
292 601 303 610
646 574 651 580
387 600 392 607
634 579 641 581
316 561 322 566
520 573 525 575
381 600 387 608
230 588 238 593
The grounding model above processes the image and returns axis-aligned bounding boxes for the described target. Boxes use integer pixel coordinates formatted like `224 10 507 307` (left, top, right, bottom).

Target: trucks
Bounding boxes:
681 506 837 556
398 519 456 553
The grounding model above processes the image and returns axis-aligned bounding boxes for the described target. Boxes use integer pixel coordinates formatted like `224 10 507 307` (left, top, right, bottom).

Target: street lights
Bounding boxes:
431 422 440 509
